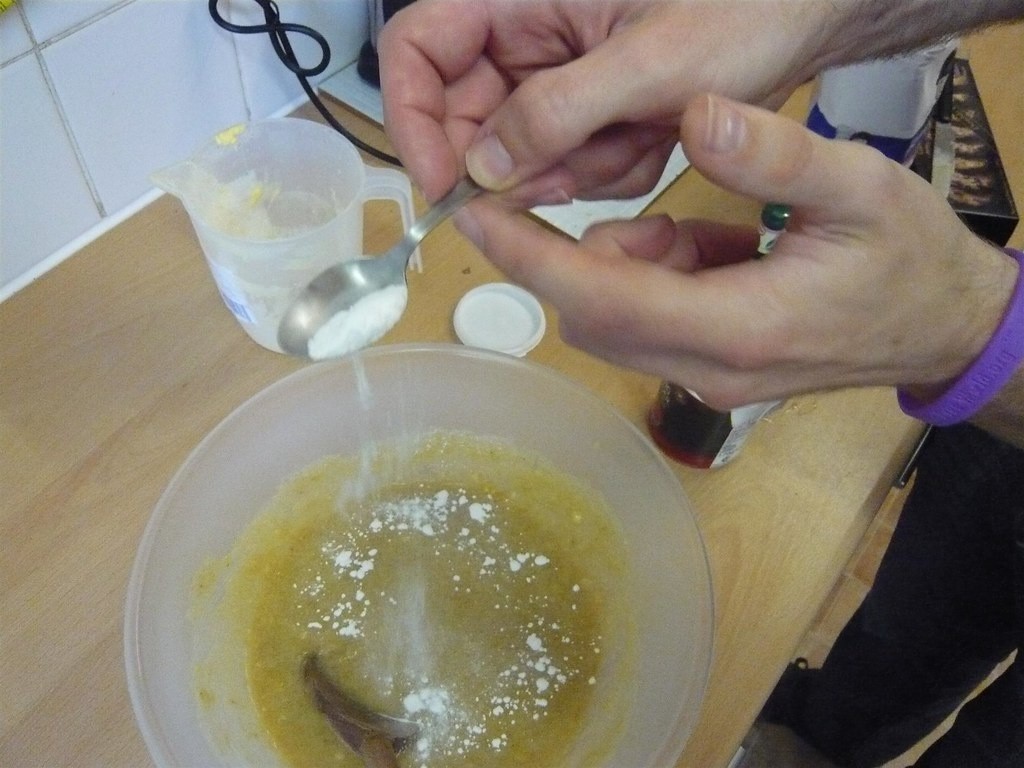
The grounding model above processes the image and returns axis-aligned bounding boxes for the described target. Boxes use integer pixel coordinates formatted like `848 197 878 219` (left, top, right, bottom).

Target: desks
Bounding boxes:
0 17 1024 767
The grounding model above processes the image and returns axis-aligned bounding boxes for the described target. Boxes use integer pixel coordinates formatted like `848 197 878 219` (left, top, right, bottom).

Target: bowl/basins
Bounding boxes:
122 342 715 768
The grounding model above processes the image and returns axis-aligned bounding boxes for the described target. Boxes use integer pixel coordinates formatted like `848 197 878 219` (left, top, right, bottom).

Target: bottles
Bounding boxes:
647 203 796 469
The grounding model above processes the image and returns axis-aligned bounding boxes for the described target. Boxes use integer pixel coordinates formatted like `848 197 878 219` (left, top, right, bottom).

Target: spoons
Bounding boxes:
278 173 485 358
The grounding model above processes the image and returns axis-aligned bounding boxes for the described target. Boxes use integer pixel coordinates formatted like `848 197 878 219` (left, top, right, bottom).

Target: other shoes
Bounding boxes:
761 656 816 724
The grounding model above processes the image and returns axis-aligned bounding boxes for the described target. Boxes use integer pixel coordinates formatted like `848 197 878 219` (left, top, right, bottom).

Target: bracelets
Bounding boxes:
896 248 1024 426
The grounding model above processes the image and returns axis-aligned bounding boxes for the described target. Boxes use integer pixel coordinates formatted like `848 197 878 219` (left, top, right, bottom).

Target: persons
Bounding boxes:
377 0 1024 768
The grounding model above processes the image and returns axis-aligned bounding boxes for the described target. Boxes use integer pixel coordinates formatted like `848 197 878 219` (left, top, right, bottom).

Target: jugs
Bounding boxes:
149 118 424 354
